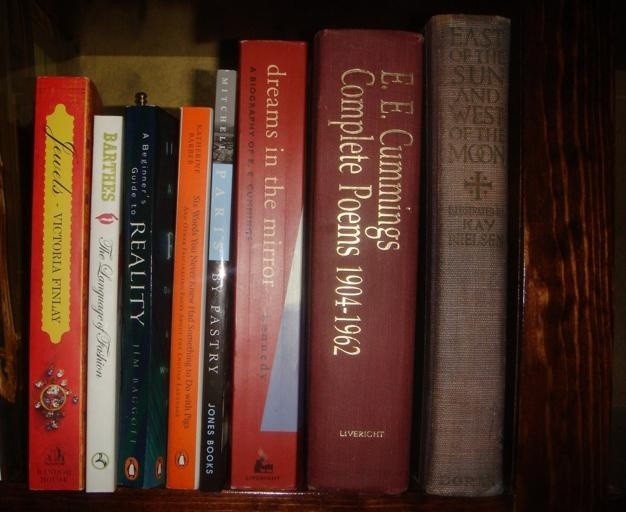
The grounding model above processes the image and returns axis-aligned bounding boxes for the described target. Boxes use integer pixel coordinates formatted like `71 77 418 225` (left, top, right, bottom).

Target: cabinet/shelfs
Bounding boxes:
0 0 626 512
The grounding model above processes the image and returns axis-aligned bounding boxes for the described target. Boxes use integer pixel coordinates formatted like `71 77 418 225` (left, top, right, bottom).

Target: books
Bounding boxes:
85 115 124 494
166 107 214 490
201 69 239 490
306 27 424 496
117 107 154 489
232 40 307 493
411 12 510 496
143 107 181 489
29 77 101 490
